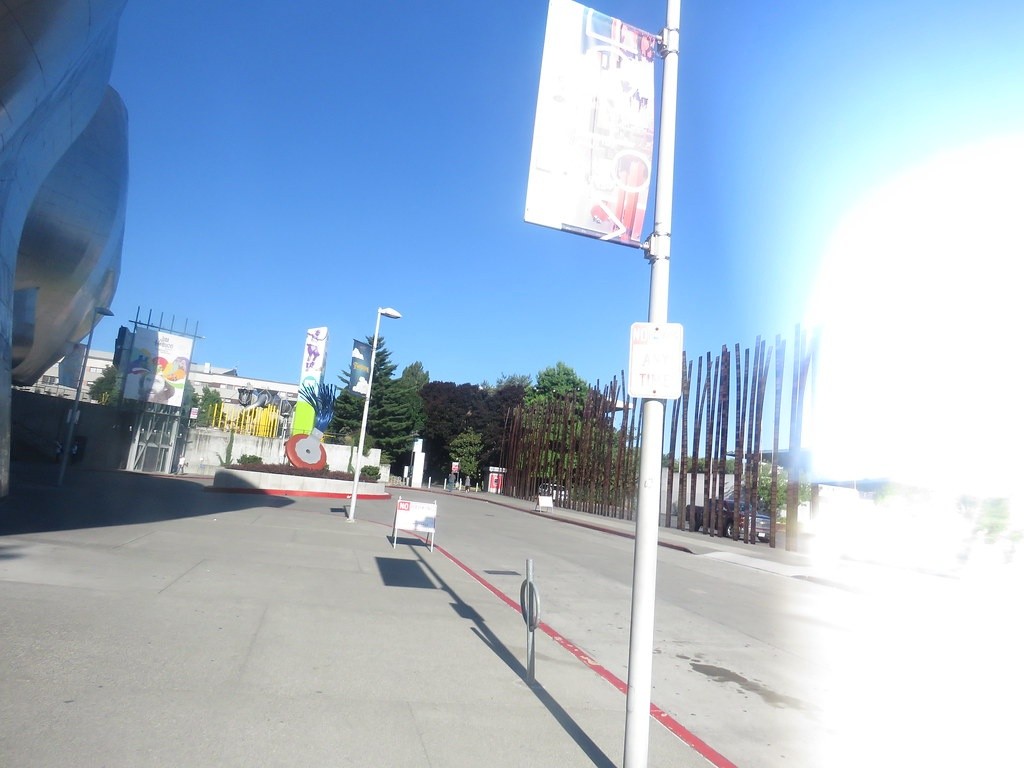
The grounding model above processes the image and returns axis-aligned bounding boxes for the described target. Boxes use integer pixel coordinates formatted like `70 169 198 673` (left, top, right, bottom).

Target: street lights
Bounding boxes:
56 305 114 491
347 307 402 523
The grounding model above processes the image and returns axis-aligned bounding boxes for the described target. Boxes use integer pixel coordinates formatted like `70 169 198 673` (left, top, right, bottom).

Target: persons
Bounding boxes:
174 454 185 474
447 470 456 492
464 476 470 493
138 371 175 404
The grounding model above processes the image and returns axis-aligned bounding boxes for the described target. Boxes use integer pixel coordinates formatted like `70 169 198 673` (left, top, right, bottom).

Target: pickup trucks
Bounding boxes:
685 499 771 543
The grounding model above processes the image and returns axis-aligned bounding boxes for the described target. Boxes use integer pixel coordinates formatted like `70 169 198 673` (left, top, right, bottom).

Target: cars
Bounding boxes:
539 483 568 501
